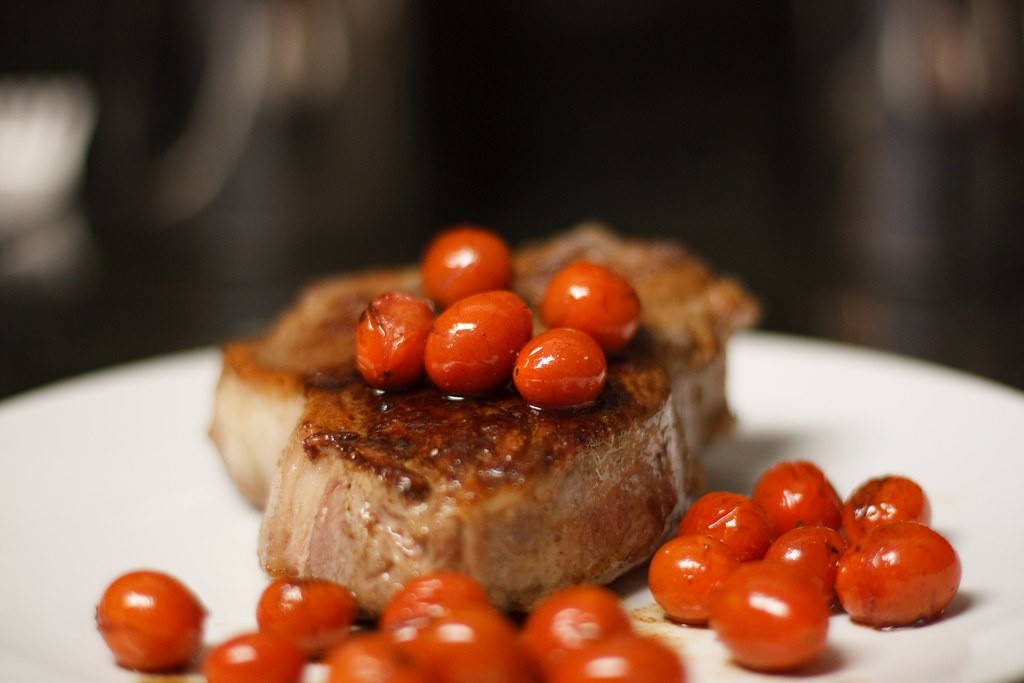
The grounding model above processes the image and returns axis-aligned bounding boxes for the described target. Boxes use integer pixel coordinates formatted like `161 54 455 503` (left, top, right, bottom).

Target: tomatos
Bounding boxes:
352 226 640 407
649 458 964 671
93 572 686 683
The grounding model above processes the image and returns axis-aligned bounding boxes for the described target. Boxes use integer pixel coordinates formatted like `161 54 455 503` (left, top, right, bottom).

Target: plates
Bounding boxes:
0 331 1024 683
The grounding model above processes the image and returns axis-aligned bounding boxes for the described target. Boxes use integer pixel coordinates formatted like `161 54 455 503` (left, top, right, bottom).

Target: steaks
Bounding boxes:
206 222 745 616
